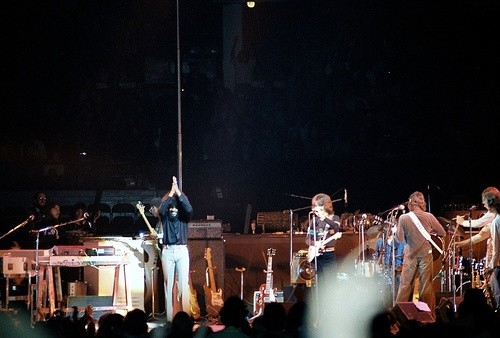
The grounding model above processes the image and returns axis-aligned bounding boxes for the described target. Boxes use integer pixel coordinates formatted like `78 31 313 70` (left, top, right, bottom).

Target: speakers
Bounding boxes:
391 302 436 324
291 253 316 284
65 296 113 323
173 239 225 320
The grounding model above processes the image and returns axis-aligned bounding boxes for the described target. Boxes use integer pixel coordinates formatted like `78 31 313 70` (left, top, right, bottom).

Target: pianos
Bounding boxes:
38 244 128 266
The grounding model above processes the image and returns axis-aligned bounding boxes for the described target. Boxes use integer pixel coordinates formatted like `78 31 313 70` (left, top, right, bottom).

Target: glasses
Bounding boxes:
36 197 46 200
148 206 153 213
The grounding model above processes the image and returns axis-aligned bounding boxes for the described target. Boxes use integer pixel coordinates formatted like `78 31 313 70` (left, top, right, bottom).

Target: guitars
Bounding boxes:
204 247 225 315
371 215 445 262
308 232 343 263
257 248 279 313
136 199 164 253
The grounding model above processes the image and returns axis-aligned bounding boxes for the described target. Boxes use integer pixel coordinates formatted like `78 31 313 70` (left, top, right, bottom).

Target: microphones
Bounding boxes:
344 189 347 203
392 205 405 212
26 215 34 223
468 205 476 213
84 213 92 227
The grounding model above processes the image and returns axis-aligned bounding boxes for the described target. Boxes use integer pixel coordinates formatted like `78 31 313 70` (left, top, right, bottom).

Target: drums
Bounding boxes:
450 255 487 281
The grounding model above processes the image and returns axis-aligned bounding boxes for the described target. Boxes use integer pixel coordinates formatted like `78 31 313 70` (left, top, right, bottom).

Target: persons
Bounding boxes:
28 202 65 249
62 203 101 245
10 191 46 248
0 267 500 338
147 198 167 317
451 223 494 269
159 176 194 324
455 186 500 227
373 219 407 303
305 193 340 281
393 191 446 303
483 191 500 312
